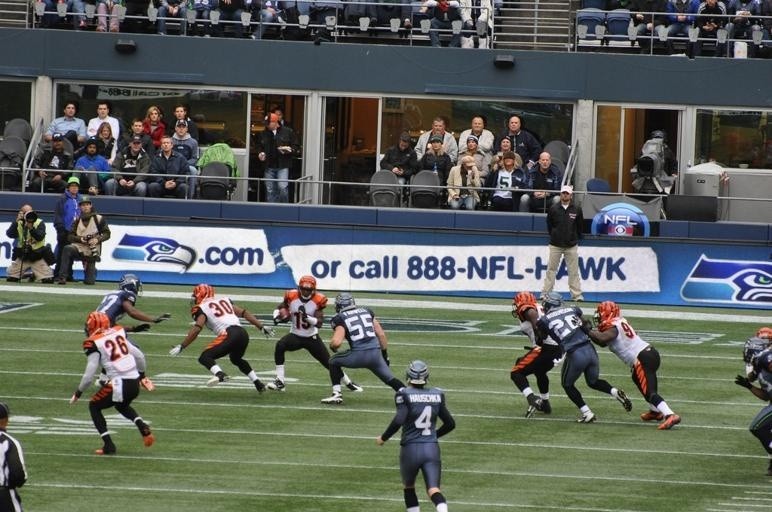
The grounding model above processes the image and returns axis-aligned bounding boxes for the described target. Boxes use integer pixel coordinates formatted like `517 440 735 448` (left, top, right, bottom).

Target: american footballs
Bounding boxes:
279 307 290 321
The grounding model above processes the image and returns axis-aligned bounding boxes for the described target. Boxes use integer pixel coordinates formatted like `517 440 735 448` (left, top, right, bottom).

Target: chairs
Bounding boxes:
408 171 441 208
5 119 31 143
608 11 632 44
576 8 605 46
549 157 565 178
198 161 230 200
0 136 26 170
369 171 403 208
587 178 611 195
544 140 571 165
188 165 197 200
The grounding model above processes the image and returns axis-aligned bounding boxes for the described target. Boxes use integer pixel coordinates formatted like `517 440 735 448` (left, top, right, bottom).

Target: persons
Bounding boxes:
170 283 276 395
695 0 728 58
510 291 563 418
267 275 364 393
377 0 411 29
423 0 462 47
457 135 491 187
250 0 287 39
414 116 457 163
35 0 72 30
72 0 97 31
95 273 172 349
729 0 764 58
629 1 673 56
536 292 632 424
485 150 525 211
94 0 126 32
746 327 772 381
488 136 523 172
143 106 164 147
415 135 451 184
6 204 55 283
88 102 120 139
651 127 677 176
345 0 378 28
74 140 111 195
54 176 83 281
216 0 248 39
734 336 772 476
447 156 483 210
460 0 493 49
43 104 86 142
148 135 190 199
573 300 681 430
82 122 118 166
458 116 494 153
279 1 313 42
0 402 28 512
376 360 456 512
254 112 300 202
380 132 418 185
321 291 406 404
122 119 152 153
519 153 563 212
171 119 198 198
188 0 215 38
57 195 111 285
31 132 71 193
169 104 199 142
273 108 287 127
157 0 188 37
507 116 541 169
540 185 585 301
105 136 152 197
666 0 700 61
70 311 156 455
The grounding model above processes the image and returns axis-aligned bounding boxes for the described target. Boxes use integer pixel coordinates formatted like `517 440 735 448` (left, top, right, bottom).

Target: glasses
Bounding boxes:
54 139 61 141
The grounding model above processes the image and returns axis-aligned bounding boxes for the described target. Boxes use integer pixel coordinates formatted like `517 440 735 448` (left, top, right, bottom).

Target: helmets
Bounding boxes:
511 291 537 318
757 327 772 340
743 336 769 363
189 283 215 305
592 300 620 328
298 275 317 301
118 273 143 296
84 311 111 336
539 292 563 315
334 292 355 314
405 360 429 386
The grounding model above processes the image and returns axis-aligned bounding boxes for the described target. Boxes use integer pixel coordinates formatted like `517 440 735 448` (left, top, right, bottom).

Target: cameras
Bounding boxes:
21 211 37 223
637 137 666 177
83 234 102 262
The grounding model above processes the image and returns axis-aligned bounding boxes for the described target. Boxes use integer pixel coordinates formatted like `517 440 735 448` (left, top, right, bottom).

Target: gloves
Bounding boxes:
141 376 156 392
153 312 171 323
580 319 592 334
383 353 390 366
734 375 752 389
132 323 151 333
169 343 184 357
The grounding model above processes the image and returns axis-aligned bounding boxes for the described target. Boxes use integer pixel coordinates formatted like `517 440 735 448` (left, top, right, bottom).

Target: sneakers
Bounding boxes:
575 410 596 424
95 443 116 454
346 381 364 392
142 432 153 448
267 378 286 392
536 402 552 414
256 382 266 392
616 389 632 412
658 414 682 429
525 395 543 418
206 372 230 387
320 391 343 404
641 412 663 421
574 294 585 302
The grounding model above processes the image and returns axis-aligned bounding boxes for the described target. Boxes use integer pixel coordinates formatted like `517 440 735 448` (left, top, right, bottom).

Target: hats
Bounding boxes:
0 402 10 418
132 134 142 144
461 155 476 166
651 130 664 138
466 135 479 143
500 135 511 142
52 132 63 141
560 185 573 195
175 119 188 127
263 112 278 125
503 151 515 159
399 129 412 142
430 134 443 144
67 176 81 186
77 195 91 205
